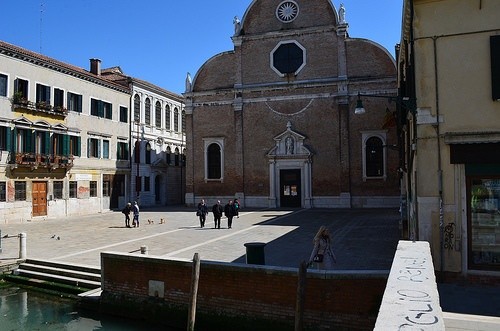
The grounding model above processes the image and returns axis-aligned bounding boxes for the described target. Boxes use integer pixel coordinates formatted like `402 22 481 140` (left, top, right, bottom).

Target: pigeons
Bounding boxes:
3 234 9 239
57 236 60 240
51 234 55 238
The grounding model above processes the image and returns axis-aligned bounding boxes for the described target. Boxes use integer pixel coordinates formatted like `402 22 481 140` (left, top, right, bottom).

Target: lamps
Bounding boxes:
354 92 398 114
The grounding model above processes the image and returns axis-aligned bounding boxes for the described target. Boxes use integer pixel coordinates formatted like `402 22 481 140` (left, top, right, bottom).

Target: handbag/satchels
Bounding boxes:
196 210 202 216
314 254 324 262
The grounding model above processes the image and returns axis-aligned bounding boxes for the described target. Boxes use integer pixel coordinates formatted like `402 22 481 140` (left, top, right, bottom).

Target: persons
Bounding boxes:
224 200 237 229
233 199 240 218
124 202 132 227
309 225 337 270
212 199 223 229
233 16 240 34
132 201 139 227
338 3 345 23
198 199 208 228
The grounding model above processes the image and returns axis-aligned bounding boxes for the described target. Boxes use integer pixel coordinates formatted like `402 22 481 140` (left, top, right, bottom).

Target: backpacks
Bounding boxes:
235 203 238 209
122 207 126 214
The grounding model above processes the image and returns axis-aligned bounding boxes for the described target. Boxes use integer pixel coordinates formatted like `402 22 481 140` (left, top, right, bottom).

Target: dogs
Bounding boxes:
161 218 166 224
148 220 154 225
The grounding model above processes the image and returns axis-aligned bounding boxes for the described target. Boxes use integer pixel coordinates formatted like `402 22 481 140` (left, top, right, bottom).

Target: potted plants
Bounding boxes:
37 100 52 110
13 91 29 105
53 104 67 113
